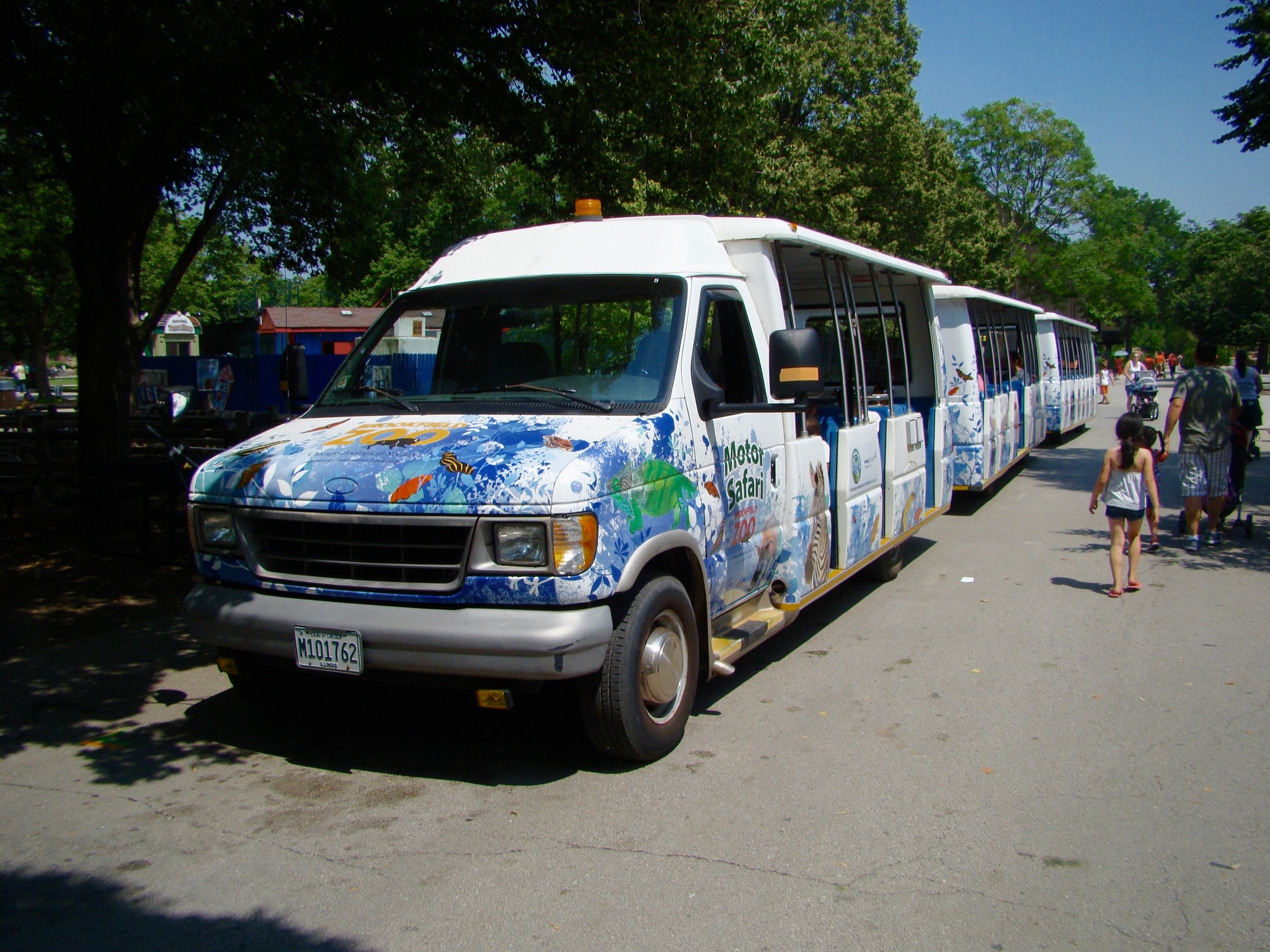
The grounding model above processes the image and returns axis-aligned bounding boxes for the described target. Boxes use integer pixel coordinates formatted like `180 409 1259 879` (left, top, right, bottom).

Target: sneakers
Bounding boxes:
1185 537 1200 551
1205 533 1223 544
1147 538 1159 551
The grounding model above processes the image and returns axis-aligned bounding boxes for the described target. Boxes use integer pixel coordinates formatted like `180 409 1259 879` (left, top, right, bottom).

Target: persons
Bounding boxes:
1088 412 1161 598
1114 356 1123 381
978 369 990 392
1099 362 1114 405
1006 349 1030 384
1144 350 1185 380
1123 353 1148 416
1231 348 1263 462
1124 427 1170 552
1162 342 1243 553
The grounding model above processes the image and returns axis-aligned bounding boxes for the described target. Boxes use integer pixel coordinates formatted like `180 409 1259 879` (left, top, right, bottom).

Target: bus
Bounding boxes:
176 198 1098 768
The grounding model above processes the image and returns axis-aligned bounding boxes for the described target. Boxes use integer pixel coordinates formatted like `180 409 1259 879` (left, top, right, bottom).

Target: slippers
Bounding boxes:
1108 585 1124 597
1127 581 1141 589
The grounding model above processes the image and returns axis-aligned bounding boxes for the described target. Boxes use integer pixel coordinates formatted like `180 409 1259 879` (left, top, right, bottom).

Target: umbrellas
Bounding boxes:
1114 350 1128 356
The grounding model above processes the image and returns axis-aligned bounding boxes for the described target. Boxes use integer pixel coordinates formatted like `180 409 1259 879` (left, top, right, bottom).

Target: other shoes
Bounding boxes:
1251 447 1260 460
1245 451 1252 462
1098 401 1110 404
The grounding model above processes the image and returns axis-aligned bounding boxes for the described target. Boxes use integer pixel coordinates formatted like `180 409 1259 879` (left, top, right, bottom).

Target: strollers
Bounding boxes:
1248 395 1263 460
1177 420 1253 539
1130 371 1159 421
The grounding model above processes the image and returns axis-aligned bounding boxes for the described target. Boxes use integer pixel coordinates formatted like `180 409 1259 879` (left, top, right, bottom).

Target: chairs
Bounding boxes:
628 329 669 377
480 340 548 387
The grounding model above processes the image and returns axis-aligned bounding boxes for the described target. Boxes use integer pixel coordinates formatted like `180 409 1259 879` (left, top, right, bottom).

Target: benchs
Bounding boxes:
815 394 937 541
978 379 1026 456
